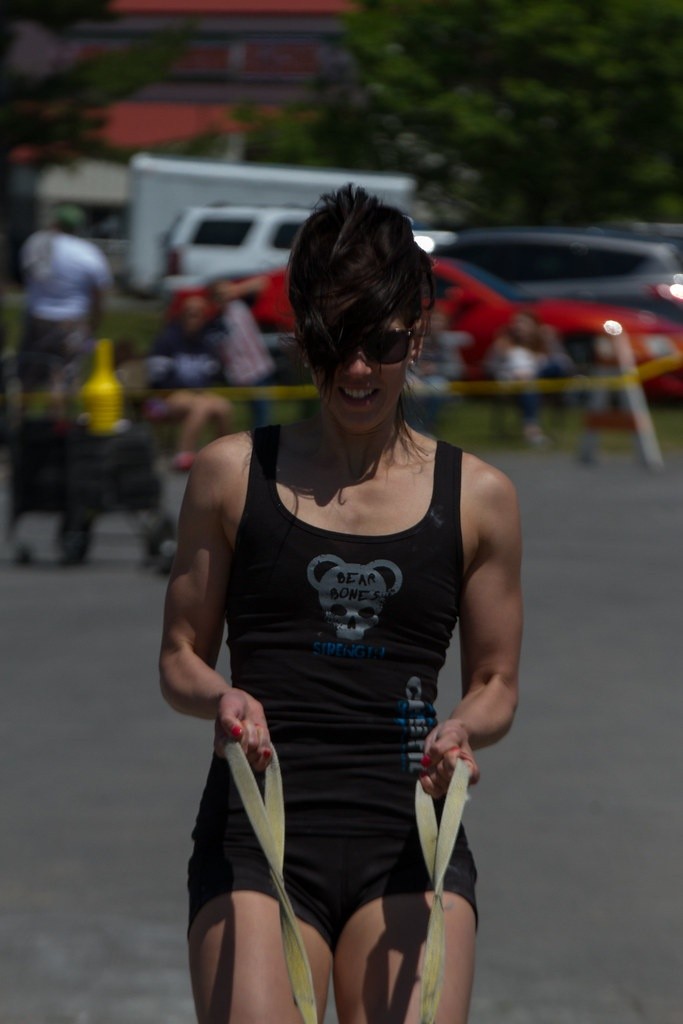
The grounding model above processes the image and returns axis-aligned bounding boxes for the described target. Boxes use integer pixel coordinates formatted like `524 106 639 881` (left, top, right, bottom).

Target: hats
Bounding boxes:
54 204 85 229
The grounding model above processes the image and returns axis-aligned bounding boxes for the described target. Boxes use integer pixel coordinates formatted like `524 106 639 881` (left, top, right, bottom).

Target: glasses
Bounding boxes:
297 315 419 370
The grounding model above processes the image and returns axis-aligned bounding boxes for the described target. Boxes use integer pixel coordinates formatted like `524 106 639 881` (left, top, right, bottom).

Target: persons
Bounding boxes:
119 268 637 447
6 335 179 570
155 179 521 1021
13 200 116 391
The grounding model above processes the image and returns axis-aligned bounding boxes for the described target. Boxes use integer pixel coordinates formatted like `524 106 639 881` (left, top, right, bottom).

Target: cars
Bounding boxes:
428 226 682 329
153 248 683 419
155 205 305 299
